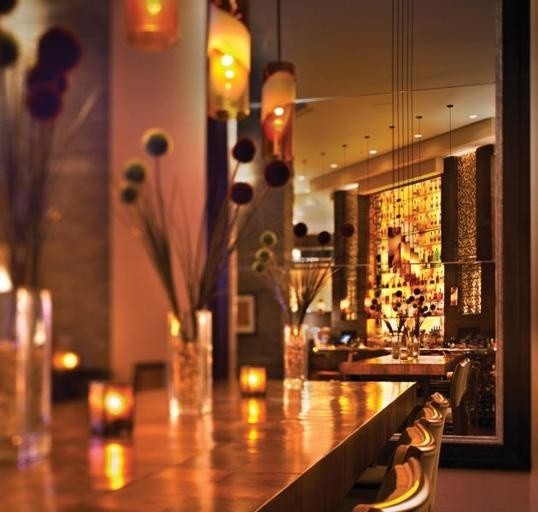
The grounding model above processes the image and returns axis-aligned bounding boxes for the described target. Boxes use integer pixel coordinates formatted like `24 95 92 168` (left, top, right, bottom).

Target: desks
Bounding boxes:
339 353 467 403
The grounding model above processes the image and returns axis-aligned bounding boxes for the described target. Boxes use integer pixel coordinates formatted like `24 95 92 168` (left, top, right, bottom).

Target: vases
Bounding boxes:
171 343 214 417
390 340 420 360
282 324 308 392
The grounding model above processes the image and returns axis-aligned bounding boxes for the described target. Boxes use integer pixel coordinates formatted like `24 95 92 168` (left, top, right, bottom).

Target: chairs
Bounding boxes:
350 356 473 512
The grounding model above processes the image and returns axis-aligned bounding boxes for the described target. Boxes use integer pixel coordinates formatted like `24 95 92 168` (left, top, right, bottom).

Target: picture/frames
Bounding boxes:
236 289 259 339
